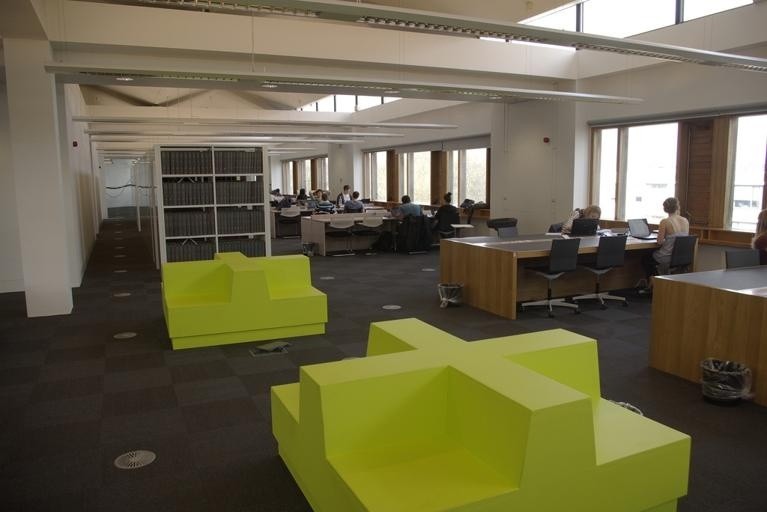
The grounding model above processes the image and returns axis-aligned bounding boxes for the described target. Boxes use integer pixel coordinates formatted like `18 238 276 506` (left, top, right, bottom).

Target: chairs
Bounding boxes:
162 252 328 350
569 234 629 310
265 195 651 257
270 317 690 511
722 248 760 269
637 236 699 298
518 238 581 319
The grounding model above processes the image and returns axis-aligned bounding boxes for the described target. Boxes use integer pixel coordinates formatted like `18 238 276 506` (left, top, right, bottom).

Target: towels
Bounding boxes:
700 357 749 406
438 283 463 307
302 243 317 256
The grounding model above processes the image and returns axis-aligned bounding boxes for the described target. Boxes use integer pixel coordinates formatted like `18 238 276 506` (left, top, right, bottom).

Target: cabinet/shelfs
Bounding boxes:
131 144 272 273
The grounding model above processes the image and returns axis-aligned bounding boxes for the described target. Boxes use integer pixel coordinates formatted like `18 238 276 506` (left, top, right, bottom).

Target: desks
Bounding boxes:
439 229 698 318
648 265 767 409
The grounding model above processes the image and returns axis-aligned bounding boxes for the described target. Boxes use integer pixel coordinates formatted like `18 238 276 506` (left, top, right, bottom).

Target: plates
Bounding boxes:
131 144 272 273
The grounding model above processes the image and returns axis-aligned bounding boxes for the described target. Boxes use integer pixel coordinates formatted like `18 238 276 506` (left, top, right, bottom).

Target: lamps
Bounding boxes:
477 36 576 53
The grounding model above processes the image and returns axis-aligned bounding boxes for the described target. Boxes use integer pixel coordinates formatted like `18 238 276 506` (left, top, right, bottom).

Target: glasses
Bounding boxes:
477 36 576 53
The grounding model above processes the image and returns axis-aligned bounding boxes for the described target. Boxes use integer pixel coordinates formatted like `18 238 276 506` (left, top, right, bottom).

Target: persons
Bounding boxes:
270 185 363 214
561 205 600 235
392 196 422 217
751 209 767 250
642 199 690 289
433 192 456 231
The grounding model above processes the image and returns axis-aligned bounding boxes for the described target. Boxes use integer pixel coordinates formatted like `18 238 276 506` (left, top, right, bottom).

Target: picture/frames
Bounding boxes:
648 265 767 409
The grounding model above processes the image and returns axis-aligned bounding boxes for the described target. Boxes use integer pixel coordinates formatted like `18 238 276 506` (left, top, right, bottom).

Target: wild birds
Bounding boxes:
564 218 600 237
627 218 658 241
